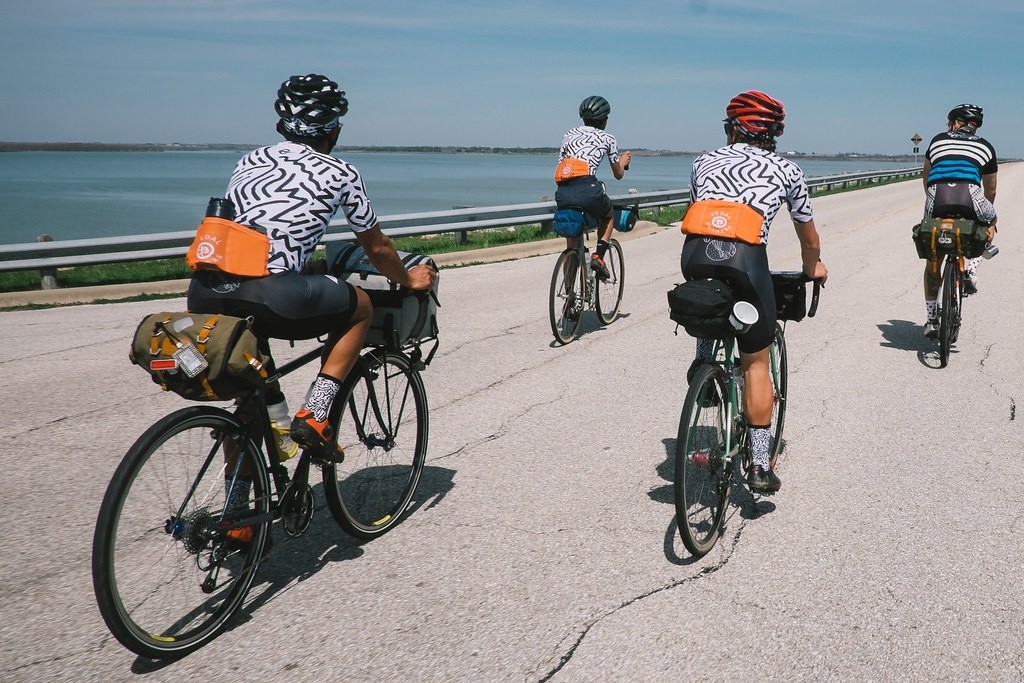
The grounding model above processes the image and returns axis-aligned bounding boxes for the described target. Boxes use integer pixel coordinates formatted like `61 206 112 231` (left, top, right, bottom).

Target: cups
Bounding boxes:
205 196 235 221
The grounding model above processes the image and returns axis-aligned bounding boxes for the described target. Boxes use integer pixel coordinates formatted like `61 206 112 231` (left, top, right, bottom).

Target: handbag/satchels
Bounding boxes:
912 215 989 259
128 310 272 400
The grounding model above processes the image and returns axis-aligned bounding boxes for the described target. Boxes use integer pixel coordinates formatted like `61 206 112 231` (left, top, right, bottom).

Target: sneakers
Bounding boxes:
589 253 611 280
226 520 275 551
289 410 346 463
562 303 579 320
748 465 781 493
686 360 720 409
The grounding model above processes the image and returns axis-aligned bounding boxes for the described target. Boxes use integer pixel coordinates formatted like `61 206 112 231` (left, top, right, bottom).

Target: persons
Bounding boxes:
555 96 632 303
680 89 828 492
188 74 436 551
922 103 998 339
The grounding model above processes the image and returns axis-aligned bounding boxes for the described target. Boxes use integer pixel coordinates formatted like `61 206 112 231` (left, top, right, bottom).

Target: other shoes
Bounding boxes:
962 272 978 296
924 322 937 340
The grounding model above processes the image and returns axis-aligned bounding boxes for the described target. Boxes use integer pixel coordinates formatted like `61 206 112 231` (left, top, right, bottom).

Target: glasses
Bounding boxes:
722 122 737 140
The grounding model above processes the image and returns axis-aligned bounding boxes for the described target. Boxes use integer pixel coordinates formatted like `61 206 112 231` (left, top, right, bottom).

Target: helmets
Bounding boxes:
948 106 985 126
271 73 348 140
728 93 788 138
578 97 610 121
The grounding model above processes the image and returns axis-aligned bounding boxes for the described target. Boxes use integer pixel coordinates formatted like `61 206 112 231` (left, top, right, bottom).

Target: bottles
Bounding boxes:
299 380 316 410
265 388 298 457
724 355 743 389
584 246 591 278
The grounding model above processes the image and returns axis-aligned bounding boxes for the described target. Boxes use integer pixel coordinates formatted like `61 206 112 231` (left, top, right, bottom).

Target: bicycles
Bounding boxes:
912 204 999 367
666 266 826 555
92 253 442 655
548 199 642 346
